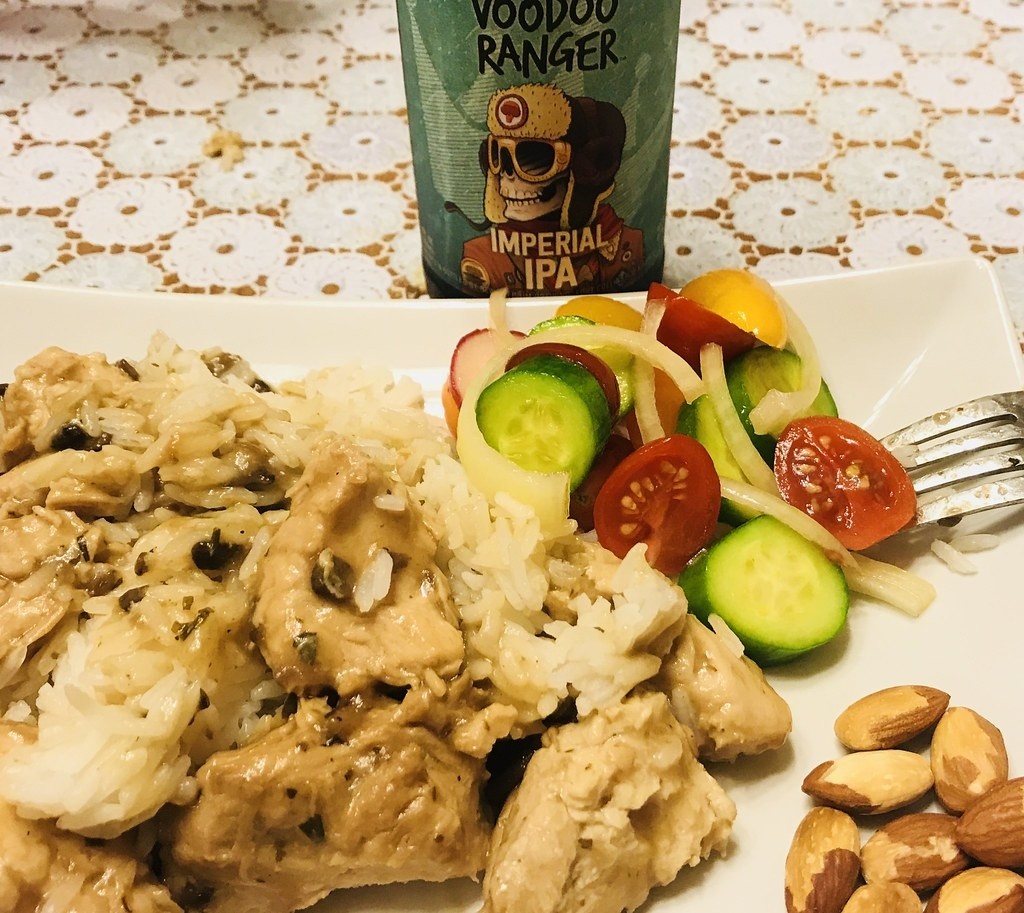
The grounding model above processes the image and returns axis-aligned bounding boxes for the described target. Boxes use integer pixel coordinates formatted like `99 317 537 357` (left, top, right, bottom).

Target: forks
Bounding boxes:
876 389 1023 531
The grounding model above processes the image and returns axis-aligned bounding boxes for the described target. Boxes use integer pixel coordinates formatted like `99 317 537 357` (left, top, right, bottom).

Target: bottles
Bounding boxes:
396 0 681 300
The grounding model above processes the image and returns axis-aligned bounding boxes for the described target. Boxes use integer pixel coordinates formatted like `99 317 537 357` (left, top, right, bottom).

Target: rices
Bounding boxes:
0 340 792 912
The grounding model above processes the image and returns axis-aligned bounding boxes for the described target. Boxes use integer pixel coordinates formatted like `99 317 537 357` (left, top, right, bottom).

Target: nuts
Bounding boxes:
783 685 1024 913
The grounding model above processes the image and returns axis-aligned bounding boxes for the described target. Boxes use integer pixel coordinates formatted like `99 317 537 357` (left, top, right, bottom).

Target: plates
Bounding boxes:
0 258 1022 913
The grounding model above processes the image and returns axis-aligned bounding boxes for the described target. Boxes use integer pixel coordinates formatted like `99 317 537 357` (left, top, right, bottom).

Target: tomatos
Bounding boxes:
508 279 915 592
